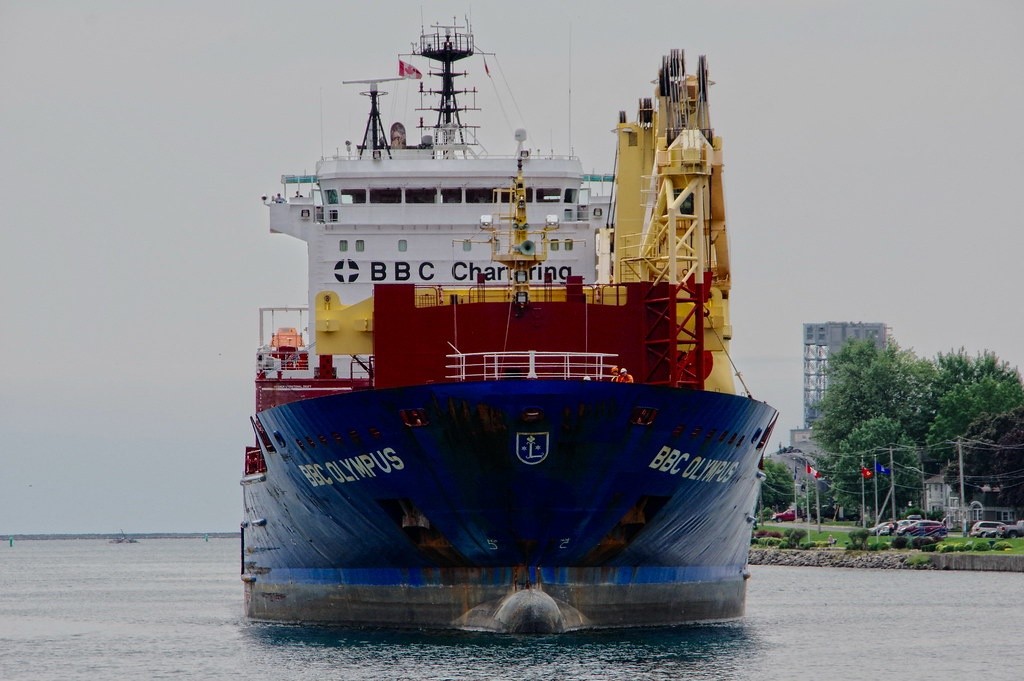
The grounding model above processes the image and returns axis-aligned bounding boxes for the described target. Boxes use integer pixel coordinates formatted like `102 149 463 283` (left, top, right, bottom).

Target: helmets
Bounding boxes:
620 369 627 372
612 367 618 372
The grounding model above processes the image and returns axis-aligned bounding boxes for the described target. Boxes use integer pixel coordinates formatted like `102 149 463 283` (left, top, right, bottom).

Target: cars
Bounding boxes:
897 520 947 537
772 509 795 523
969 521 1007 538
869 522 890 536
879 520 918 535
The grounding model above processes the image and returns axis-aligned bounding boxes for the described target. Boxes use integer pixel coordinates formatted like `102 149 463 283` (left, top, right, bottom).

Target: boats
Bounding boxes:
239 25 778 634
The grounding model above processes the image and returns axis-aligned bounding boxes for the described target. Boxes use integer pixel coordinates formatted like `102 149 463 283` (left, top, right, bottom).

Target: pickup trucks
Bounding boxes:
997 520 1024 537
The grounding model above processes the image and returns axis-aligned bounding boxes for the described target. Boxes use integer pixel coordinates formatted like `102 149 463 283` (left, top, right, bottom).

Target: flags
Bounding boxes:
807 464 821 479
863 467 871 479
876 462 889 475
793 467 799 479
399 59 421 79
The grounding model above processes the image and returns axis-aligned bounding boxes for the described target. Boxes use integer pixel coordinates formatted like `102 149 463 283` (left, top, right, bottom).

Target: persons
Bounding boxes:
293 192 302 199
889 519 898 536
828 534 834 542
274 193 284 203
611 368 633 383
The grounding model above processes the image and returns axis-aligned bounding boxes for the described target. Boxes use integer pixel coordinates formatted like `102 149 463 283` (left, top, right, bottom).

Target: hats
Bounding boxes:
277 194 280 196
